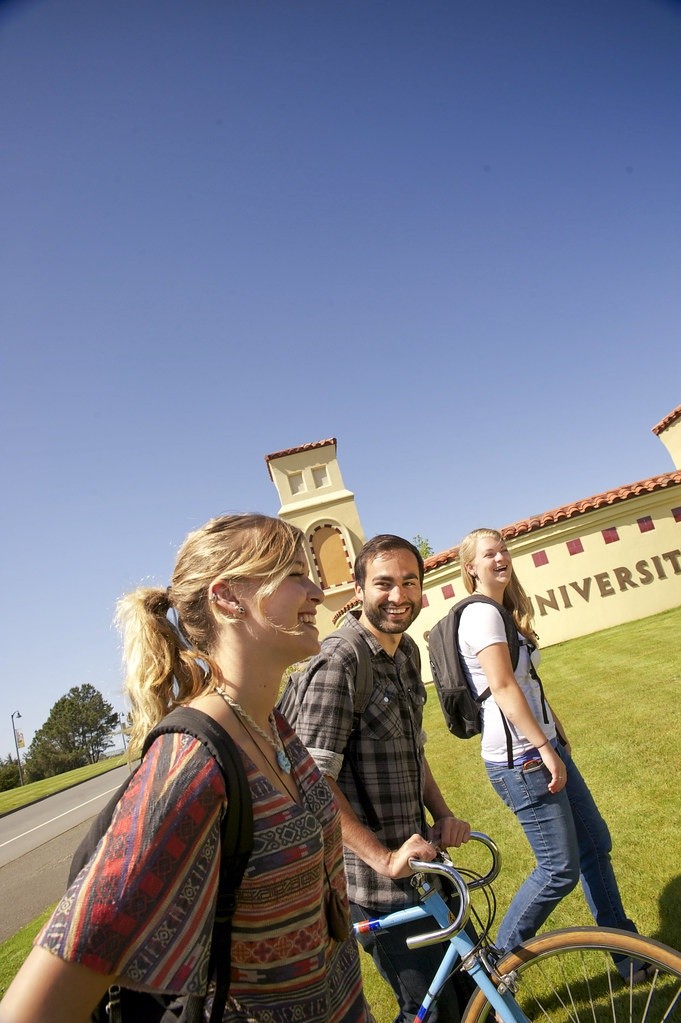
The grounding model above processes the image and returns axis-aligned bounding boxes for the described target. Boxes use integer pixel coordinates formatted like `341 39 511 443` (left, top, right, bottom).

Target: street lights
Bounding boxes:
118 710 128 751
11 710 25 786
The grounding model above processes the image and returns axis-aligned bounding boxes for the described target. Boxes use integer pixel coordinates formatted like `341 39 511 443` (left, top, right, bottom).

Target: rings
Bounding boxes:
558 776 563 779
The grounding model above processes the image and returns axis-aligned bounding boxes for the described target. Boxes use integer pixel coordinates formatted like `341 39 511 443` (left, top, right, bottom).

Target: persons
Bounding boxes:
457 527 669 998
289 534 494 1023
1 510 372 1022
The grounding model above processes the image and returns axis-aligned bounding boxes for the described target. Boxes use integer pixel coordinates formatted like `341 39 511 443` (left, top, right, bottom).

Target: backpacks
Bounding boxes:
67 707 254 1022
424 595 520 739
275 626 428 763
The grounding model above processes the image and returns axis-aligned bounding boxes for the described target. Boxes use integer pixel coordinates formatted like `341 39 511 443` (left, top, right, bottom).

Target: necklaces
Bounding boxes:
202 674 292 774
475 588 484 597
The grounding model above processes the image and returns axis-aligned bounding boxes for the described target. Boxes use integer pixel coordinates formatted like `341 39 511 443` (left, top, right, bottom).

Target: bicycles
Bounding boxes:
345 830 681 1023
